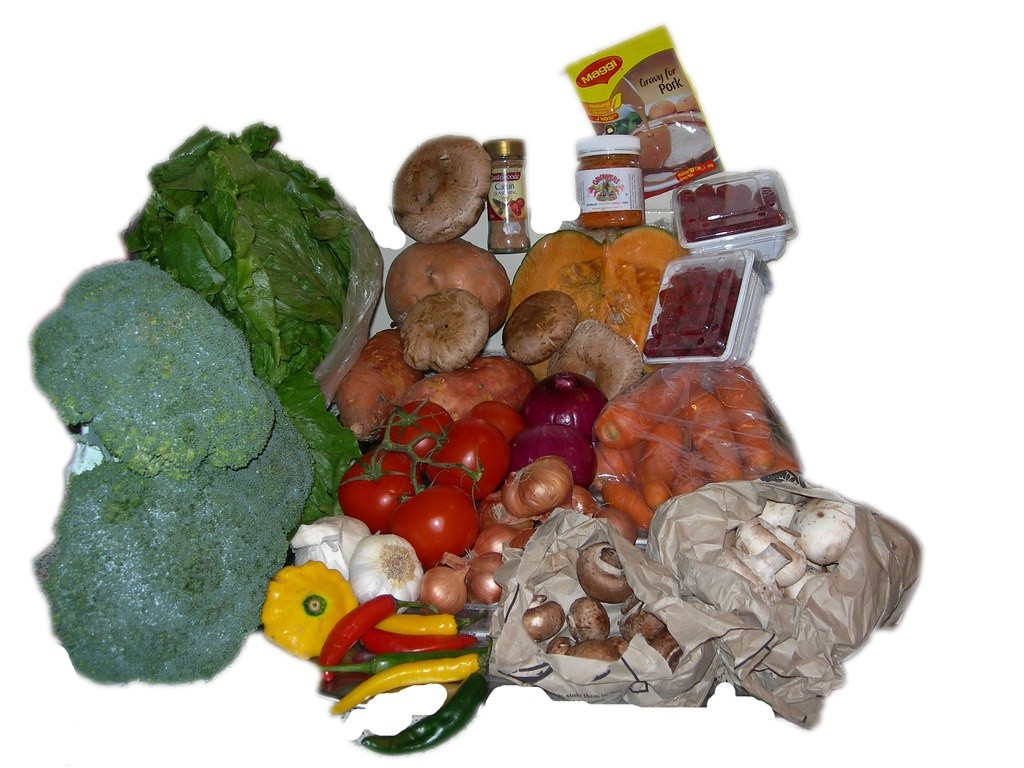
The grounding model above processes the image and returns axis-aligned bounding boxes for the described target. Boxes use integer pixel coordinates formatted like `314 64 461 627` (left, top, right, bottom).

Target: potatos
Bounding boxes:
335 329 424 441
402 356 538 418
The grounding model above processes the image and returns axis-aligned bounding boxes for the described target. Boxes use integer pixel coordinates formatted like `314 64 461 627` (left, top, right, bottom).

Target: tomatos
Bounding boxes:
337 399 526 567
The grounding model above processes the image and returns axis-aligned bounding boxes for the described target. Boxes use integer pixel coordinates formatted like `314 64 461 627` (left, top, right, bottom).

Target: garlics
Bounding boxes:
289 514 372 582
347 533 425 612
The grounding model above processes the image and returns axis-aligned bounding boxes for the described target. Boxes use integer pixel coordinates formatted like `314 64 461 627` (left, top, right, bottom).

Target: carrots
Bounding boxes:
586 364 800 533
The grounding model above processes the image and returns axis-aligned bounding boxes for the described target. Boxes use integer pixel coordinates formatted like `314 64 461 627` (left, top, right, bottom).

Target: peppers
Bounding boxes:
315 593 493 755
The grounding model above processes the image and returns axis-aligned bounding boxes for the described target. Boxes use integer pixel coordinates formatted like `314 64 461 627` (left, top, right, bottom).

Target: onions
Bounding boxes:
417 371 610 618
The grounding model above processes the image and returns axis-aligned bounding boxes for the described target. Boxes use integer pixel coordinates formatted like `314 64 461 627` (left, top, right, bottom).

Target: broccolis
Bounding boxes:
29 260 314 685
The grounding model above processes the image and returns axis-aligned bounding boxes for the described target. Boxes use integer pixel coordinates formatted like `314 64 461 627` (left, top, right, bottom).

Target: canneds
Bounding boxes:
482 137 531 254
574 134 645 231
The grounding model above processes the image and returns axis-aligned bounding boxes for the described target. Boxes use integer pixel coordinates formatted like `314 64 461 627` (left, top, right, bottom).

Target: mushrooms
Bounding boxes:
519 541 685 675
682 498 857 604
383 133 644 403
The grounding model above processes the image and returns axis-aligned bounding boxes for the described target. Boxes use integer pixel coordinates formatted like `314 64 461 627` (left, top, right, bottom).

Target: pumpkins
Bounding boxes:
504 225 693 375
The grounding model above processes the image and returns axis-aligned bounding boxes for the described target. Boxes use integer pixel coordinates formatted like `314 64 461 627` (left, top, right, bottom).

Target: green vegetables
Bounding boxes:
116 122 364 525
